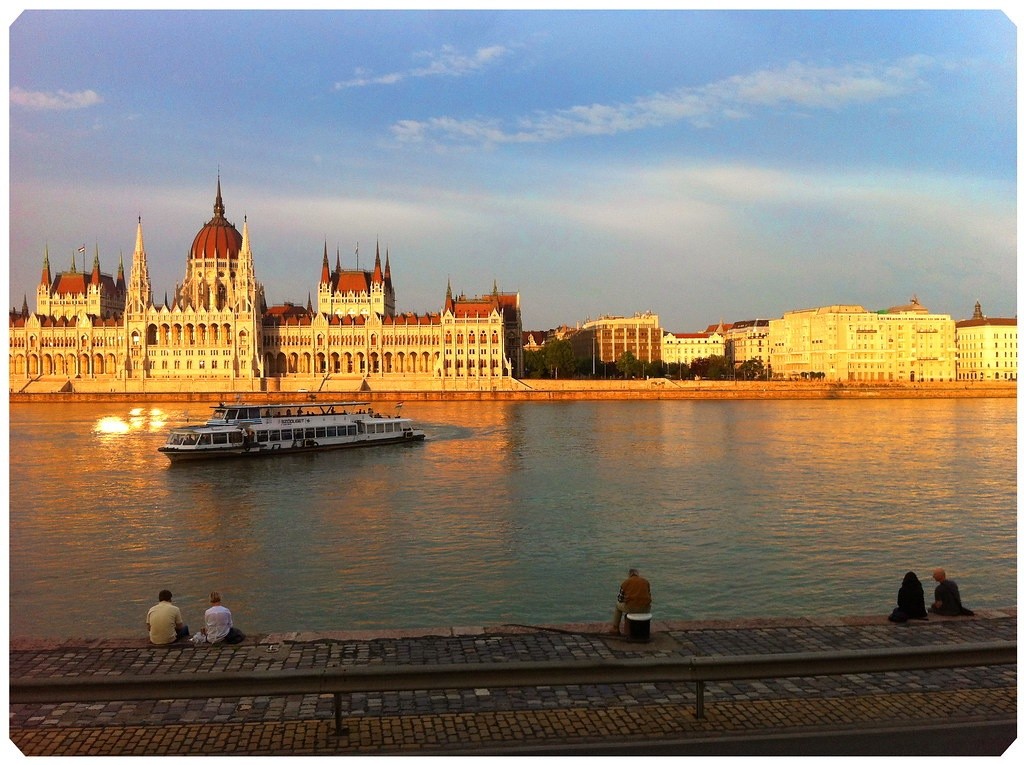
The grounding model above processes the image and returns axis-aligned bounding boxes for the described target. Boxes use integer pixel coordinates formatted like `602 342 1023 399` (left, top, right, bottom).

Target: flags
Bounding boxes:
394 401 404 408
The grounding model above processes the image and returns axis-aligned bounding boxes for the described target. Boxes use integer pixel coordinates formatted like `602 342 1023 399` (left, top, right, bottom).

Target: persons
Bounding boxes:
265 406 315 417
888 567 963 623
355 407 381 417
608 568 652 634
240 427 248 442
146 588 190 646
205 590 235 644
331 407 335 414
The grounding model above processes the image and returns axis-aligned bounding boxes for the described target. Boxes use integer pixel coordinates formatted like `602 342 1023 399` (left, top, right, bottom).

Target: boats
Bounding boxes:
158 397 426 464
